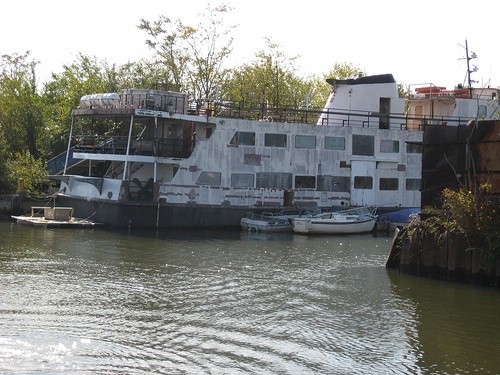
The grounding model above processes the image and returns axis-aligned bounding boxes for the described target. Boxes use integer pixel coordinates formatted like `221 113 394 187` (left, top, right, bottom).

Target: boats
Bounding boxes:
287 206 380 234
239 211 318 233
